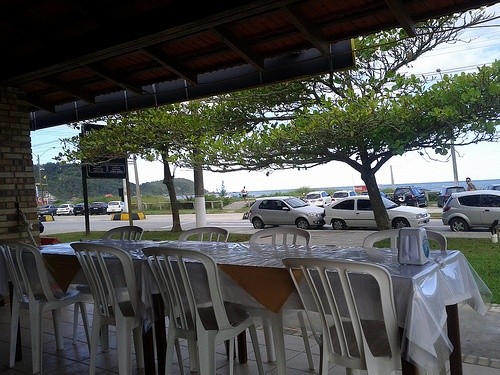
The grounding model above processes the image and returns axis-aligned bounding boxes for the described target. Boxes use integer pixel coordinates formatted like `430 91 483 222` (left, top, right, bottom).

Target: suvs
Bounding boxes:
394 186 428 208
90 202 108 215
107 201 125 215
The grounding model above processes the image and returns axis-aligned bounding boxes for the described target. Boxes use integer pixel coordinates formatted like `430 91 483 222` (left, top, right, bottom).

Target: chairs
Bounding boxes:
249 226 310 245
176 226 230 241
67 241 157 375
141 246 267 375
281 256 405 375
361 229 447 250
0 239 91 375
70 226 143 353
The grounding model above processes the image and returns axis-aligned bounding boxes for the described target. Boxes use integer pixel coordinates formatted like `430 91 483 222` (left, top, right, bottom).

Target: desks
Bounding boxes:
0 236 493 375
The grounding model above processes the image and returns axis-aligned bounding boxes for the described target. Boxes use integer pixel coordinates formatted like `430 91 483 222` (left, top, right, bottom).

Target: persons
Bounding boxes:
242 187 247 200
466 178 477 191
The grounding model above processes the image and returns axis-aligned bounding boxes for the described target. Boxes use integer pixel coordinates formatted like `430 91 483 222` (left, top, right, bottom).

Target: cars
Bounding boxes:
437 186 466 208
73 203 91 215
104 194 113 198
56 204 75 216
303 190 393 208
442 190 500 231
248 196 325 230
484 184 500 203
47 210 48 210
323 196 430 230
38 205 56 216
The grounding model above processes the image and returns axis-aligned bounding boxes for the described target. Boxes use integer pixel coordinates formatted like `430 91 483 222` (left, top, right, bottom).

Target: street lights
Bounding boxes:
32 147 56 204
